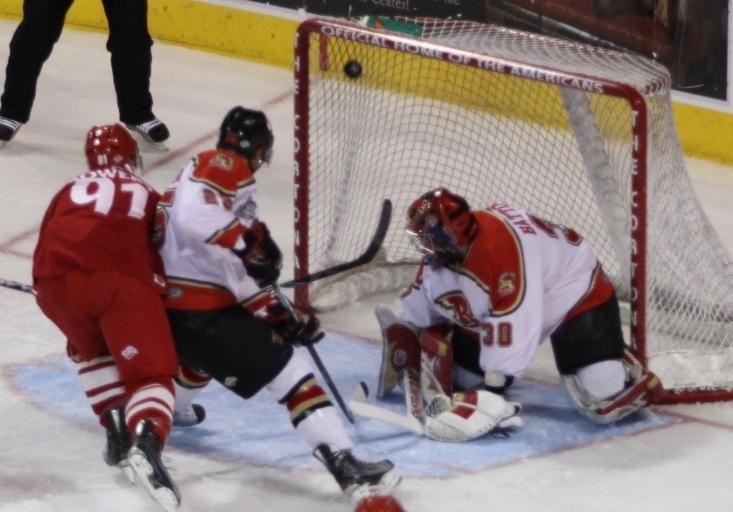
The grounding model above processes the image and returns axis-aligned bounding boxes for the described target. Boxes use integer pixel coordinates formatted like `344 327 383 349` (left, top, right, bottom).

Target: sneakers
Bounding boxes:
0 115 22 141
132 115 168 141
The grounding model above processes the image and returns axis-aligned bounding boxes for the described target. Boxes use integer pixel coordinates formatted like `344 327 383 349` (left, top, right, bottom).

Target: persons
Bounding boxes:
30 122 194 512
374 186 666 441
152 105 408 512
0 0 170 152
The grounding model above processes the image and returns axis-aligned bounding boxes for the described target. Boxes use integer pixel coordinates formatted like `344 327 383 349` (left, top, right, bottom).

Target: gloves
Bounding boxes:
241 222 282 287
264 298 325 347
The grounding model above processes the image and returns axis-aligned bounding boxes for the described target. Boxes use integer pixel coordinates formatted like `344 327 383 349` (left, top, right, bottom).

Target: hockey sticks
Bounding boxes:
307 344 369 426
278 200 392 287
350 366 429 438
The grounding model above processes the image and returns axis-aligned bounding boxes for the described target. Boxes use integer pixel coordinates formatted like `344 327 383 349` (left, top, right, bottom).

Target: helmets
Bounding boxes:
216 106 273 161
404 186 478 257
84 123 137 171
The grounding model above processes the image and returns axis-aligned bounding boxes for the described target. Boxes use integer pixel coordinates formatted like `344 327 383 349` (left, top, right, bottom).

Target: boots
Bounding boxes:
312 443 394 495
103 404 203 511
